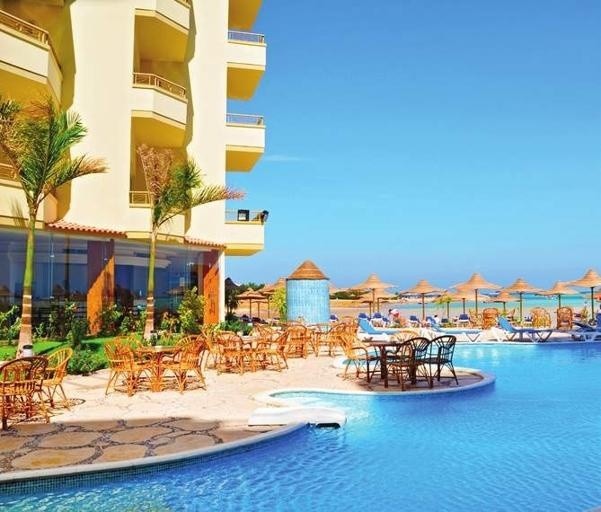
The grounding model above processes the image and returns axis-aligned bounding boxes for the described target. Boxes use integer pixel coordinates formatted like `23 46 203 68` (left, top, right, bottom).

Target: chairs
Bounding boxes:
330 312 472 333
104 333 208 395
0 355 48 432
45 347 74 412
277 318 362 360
201 323 293 375
335 329 460 390
482 305 600 343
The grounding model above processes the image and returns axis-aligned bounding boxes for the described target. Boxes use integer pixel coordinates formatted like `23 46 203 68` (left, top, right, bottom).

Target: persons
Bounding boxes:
251 210 268 223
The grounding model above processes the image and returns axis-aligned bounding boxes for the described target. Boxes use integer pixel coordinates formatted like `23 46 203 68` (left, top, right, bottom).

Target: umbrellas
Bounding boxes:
352 268 601 328
234 277 286 319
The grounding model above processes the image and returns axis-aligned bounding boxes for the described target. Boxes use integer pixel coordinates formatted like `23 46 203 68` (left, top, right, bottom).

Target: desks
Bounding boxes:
0 362 31 413
